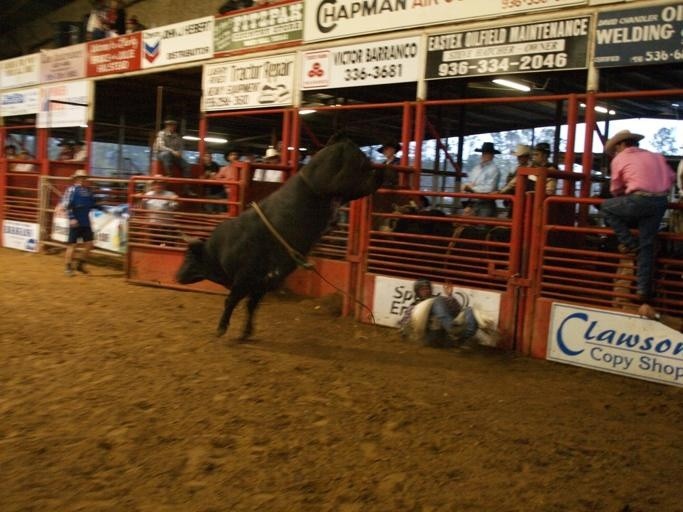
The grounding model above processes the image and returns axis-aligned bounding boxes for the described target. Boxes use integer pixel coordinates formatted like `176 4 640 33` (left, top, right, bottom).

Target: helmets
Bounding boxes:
414 277 431 298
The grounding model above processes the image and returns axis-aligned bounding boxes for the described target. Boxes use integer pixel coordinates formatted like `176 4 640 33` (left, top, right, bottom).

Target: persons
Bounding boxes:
460 141 503 228
377 194 483 252
601 130 677 321
200 137 282 185
372 137 402 189
61 168 109 279
395 278 514 356
150 117 193 181
137 171 180 250
592 163 613 202
2 127 88 171
487 142 561 222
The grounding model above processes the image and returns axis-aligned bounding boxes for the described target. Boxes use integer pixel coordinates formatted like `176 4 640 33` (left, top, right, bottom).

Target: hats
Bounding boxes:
603 129 645 154
376 138 401 154
70 169 90 177
146 174 169 183
5 143 29 156
475 142 501 154
224 145 242 161
57 138 85 147
261 148 280 159
161 114 183 122
513 143 559 157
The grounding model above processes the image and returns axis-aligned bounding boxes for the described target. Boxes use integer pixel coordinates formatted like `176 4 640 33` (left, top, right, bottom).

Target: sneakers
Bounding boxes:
617 239 637 257
63 265 88 275
638 303 660 321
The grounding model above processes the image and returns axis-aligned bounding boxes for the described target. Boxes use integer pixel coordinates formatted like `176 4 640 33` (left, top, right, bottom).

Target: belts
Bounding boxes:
635 190 668 197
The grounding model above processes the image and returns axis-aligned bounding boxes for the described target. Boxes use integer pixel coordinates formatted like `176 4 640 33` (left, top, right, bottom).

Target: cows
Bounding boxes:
174 125 400 343
